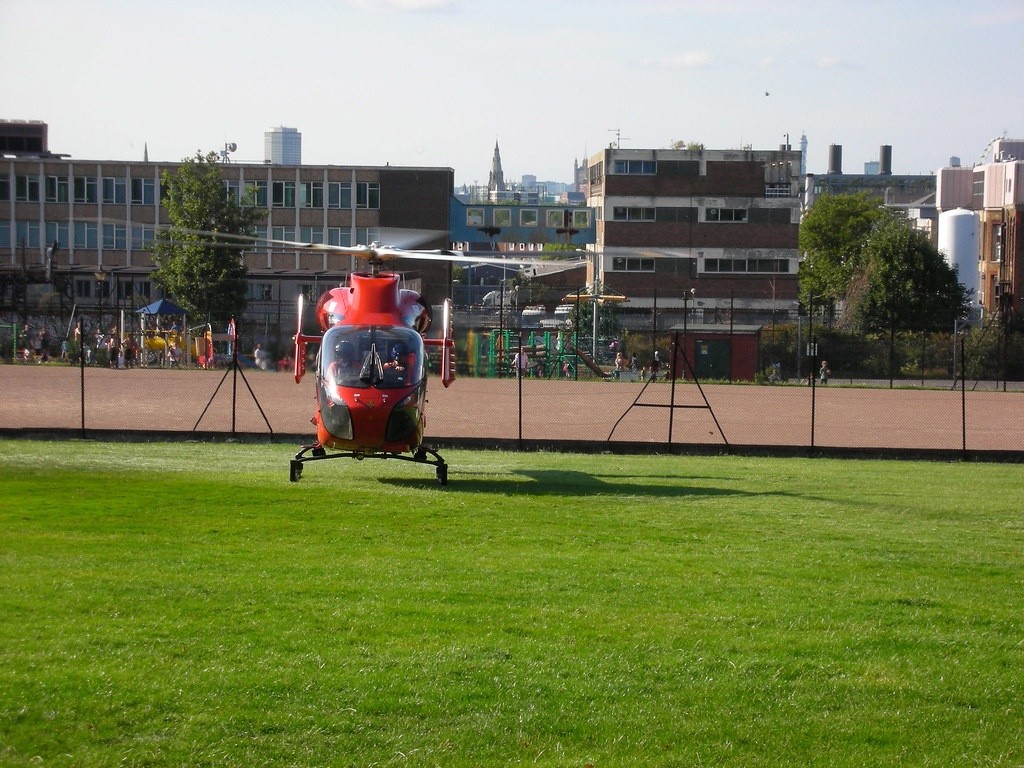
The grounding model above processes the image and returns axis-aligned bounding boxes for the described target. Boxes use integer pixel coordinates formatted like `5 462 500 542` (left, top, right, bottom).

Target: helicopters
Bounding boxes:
68 208 692 485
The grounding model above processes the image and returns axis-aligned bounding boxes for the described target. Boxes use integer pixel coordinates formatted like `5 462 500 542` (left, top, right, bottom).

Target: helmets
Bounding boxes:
391 343 409 358
335 340 354 352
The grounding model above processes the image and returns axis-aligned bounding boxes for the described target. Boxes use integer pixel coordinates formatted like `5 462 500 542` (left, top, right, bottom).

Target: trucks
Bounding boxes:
480 291 512 313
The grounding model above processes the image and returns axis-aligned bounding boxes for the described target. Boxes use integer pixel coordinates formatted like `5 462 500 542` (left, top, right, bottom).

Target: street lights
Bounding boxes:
94 264 108 332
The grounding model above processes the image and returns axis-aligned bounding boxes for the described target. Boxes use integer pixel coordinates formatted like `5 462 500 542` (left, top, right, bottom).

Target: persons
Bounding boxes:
615 352 626 377
384 343 408 372
819 361 831 385
21 315 182 369
563 362 571 380
631 353 637 372
515 348 528 377
328 340 360 380
255 344 270 370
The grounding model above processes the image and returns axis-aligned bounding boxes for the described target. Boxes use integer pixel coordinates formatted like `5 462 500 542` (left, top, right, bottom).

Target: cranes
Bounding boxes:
607 128 630 149
